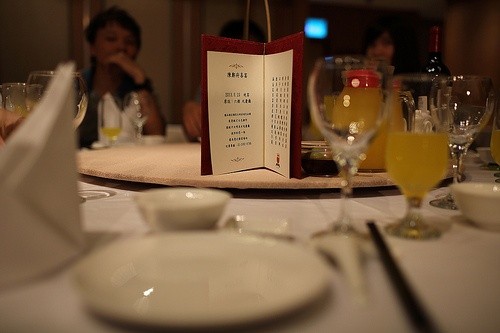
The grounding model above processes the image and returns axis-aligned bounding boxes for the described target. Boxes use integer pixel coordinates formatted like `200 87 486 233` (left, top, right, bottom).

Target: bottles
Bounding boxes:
417 25 452 110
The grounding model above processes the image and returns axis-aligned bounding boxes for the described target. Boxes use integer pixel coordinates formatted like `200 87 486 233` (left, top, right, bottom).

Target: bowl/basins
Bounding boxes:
301 151 339 177
132 188 230 230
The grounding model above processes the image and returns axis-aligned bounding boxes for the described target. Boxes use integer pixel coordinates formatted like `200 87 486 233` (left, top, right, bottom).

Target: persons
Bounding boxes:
361 19 408 72
75 4 169 149
182 15 269 144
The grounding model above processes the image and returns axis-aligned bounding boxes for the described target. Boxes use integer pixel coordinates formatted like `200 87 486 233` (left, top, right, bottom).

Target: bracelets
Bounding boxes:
131 74 154 95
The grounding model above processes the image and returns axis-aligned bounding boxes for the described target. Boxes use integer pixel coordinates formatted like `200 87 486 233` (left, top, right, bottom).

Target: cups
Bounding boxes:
97 97 122 142
5 82 41 117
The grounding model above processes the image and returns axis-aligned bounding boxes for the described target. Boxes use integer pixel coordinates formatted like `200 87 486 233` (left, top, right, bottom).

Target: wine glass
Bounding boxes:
24 70 88 204
384 77 452 240
307 56 390 243
124 92 150 144
429 75 495 210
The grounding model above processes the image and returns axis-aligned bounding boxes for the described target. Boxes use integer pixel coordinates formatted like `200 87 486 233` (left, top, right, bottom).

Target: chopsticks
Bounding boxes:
365 221 441 332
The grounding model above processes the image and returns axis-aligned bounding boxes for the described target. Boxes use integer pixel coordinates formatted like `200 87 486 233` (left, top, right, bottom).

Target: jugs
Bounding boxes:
333 70 415 173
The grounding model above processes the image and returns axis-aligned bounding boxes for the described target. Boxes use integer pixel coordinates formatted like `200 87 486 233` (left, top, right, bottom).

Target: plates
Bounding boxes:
71 230 336 329
